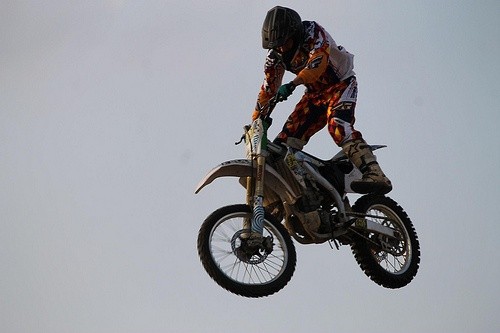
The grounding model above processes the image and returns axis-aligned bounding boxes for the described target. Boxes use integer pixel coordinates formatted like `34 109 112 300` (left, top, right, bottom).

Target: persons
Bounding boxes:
252 5 393 194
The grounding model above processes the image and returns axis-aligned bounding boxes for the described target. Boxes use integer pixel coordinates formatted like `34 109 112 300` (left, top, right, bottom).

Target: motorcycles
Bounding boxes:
195 85 421 298
